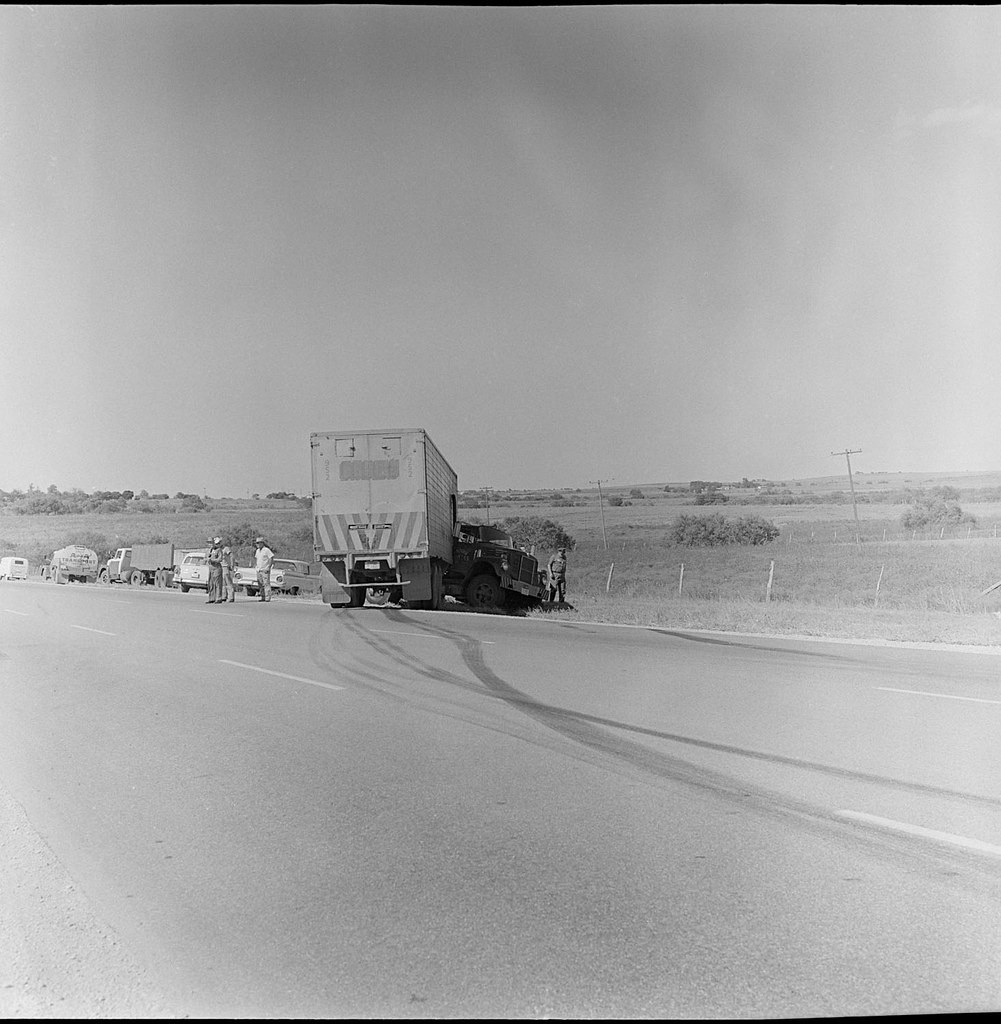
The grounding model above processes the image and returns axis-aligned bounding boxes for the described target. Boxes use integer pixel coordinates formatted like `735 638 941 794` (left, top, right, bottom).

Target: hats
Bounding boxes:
558 548 567 553
256 537 264 543
207 537 215 542
213 537 222 544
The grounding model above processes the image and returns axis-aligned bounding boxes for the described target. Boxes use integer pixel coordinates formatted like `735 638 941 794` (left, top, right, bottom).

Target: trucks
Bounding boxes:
0 557 28 580
309 428 457 610
441 519 550 609
98 544 174 588
38 545 100 584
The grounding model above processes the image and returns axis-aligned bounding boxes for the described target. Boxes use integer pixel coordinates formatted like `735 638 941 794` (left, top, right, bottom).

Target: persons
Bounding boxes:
214 538 235 602
205 537 222 604
548 547 566 604
255 537 274 602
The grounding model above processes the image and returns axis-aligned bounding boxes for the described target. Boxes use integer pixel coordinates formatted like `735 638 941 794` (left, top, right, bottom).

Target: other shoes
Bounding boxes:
206 600 215 604
257 597 264 602
215 600 222 604
222 599 226 601
230 599 234 602
265 597 270 601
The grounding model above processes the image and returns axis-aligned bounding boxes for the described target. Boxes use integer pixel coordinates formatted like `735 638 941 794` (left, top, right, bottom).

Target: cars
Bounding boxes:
171 551 211 593
233 558 319 598
